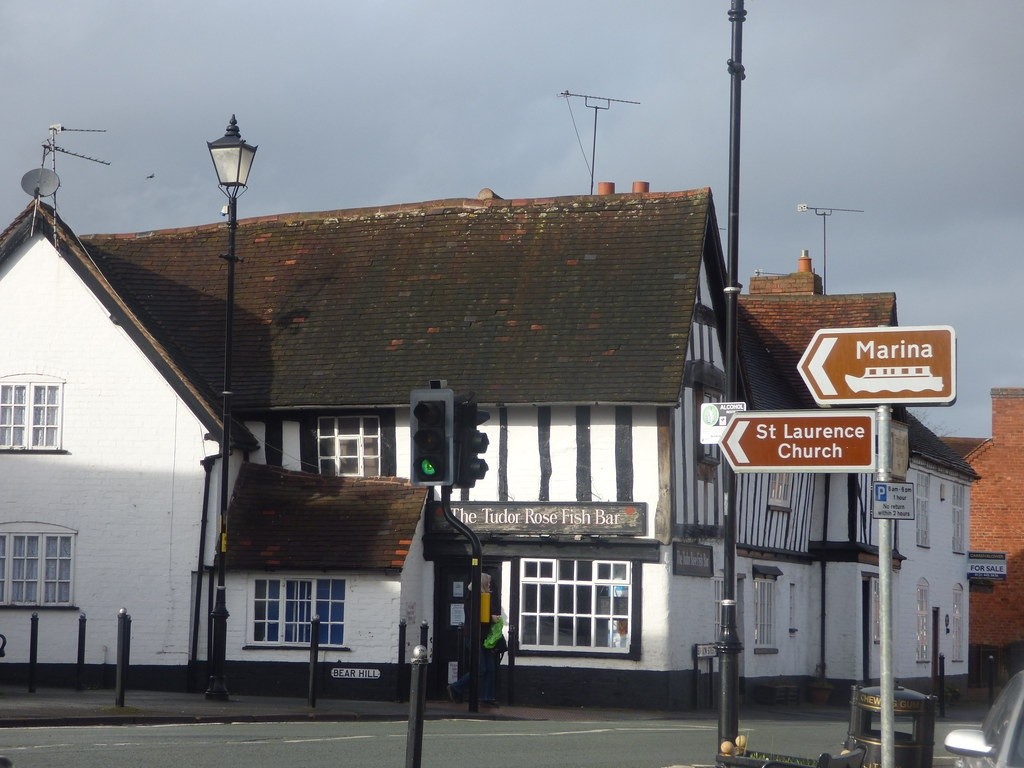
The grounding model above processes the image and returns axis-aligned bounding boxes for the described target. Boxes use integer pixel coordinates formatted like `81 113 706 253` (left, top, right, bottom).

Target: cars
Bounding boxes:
944 670 1024 768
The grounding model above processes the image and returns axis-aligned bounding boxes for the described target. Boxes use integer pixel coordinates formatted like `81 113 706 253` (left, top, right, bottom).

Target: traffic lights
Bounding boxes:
413 399 448 480
458 401 489 488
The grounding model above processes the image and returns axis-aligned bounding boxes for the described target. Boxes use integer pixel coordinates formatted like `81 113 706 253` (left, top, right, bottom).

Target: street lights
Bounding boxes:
205 114 258 703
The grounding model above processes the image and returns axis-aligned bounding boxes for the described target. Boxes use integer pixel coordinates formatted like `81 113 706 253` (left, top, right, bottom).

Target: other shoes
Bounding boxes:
482 698 500 707
447 685 455 702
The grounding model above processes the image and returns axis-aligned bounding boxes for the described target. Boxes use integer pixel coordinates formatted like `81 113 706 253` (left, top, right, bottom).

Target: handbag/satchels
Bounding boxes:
484 618 502 648
493 637 509 655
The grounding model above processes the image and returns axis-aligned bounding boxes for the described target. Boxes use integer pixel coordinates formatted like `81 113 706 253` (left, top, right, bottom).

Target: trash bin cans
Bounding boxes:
844 684 940 768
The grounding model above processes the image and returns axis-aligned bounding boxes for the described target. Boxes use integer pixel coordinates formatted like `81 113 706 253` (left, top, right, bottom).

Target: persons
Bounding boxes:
447 573 508 709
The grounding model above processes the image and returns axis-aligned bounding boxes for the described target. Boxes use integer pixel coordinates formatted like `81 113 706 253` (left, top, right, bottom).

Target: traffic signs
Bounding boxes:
797 326 958 407
717 412 876 474
